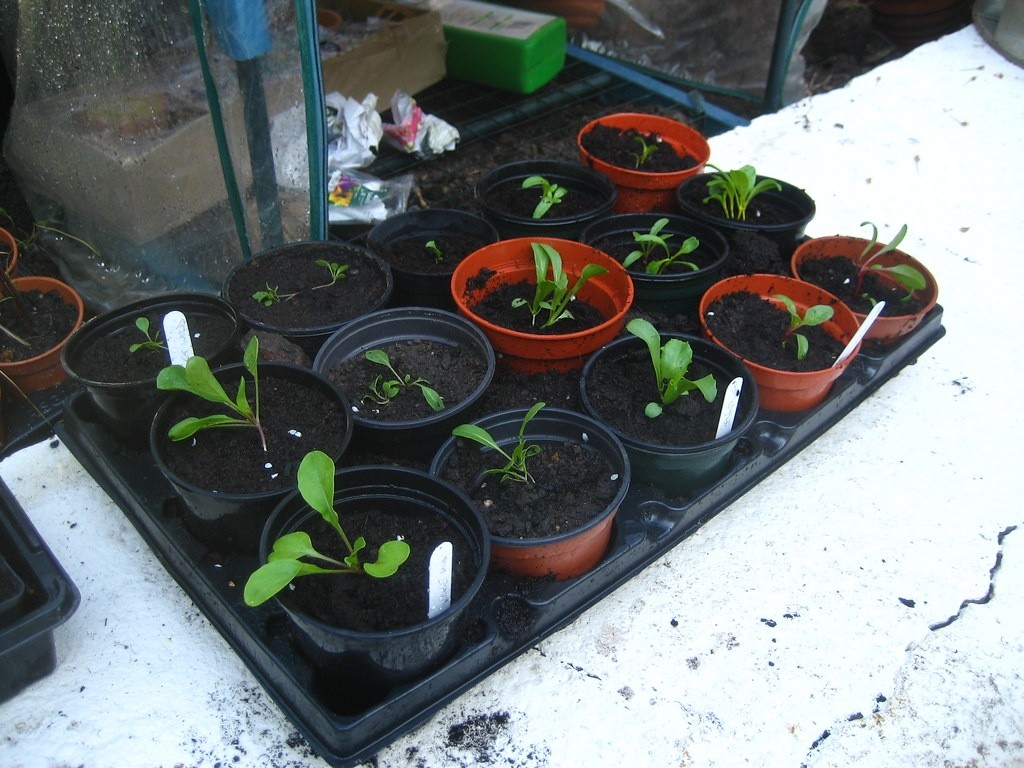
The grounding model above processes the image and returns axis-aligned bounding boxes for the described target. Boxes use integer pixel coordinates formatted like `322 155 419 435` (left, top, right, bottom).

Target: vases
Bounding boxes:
0 474 81 704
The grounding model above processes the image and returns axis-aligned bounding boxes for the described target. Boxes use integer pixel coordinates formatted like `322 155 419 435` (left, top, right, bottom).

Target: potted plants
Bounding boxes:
60 112 938 691
1 225 86 395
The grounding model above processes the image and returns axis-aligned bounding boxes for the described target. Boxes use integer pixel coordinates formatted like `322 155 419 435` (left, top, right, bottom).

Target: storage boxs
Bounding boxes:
17 1 447 247
